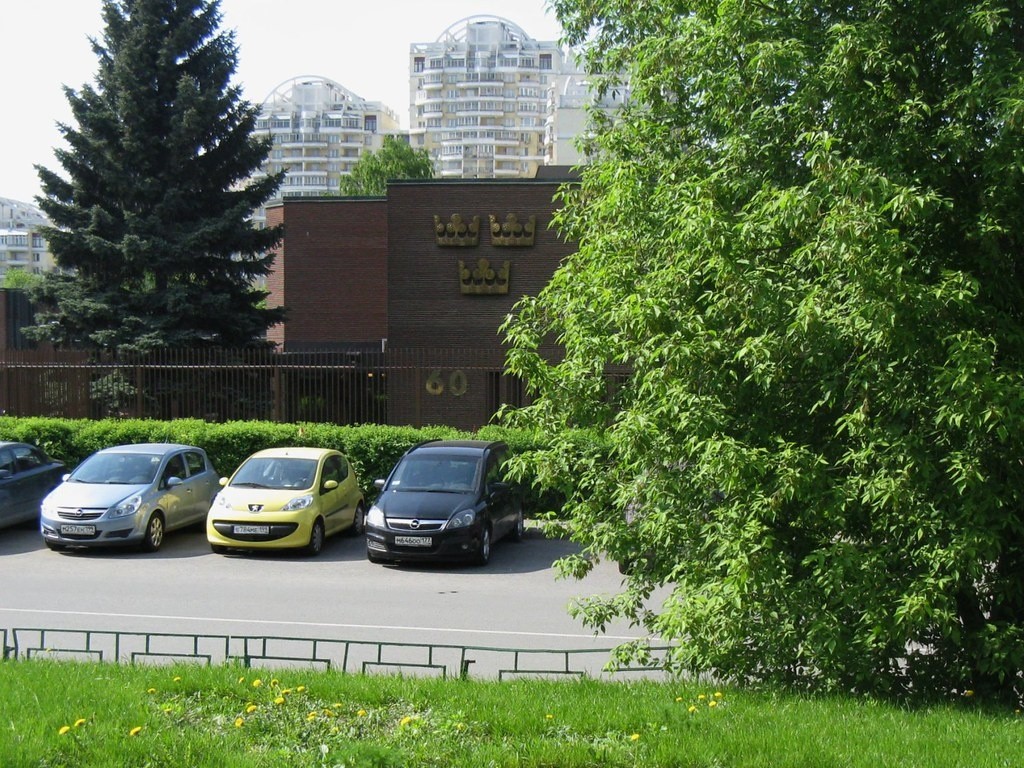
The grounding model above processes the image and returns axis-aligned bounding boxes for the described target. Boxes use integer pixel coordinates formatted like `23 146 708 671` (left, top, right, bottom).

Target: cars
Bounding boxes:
0 440 69 531
38 443 221 552
206 448 366 559
617 461 731 577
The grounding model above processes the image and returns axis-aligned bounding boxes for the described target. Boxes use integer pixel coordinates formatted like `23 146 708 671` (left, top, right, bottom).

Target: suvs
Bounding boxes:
363 439 525 568
